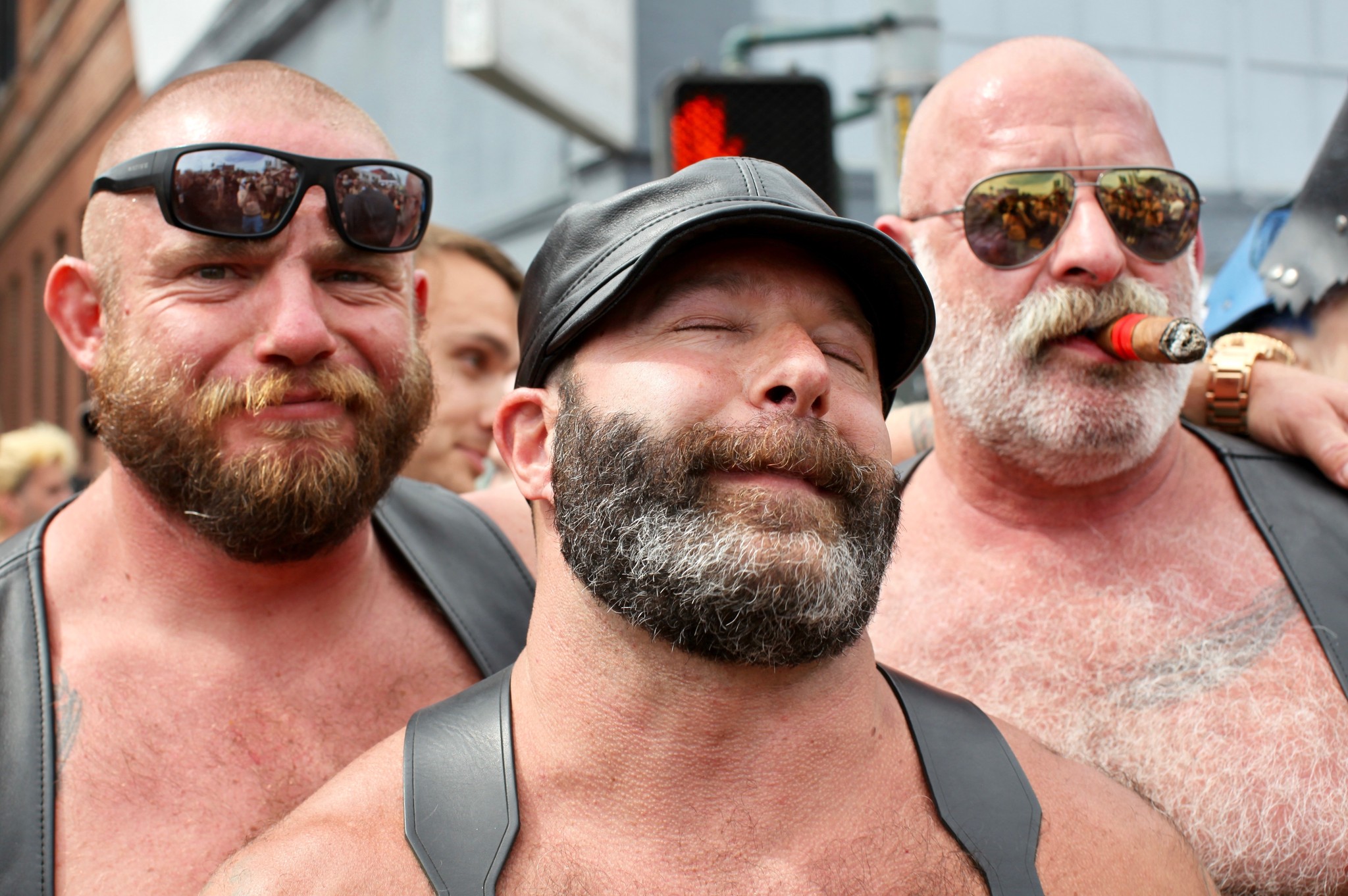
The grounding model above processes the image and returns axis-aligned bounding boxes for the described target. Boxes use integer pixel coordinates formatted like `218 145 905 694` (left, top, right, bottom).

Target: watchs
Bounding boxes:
1204 330 1297 446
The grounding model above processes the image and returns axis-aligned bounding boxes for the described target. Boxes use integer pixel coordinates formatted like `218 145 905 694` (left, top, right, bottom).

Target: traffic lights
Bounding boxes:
645 69 844 218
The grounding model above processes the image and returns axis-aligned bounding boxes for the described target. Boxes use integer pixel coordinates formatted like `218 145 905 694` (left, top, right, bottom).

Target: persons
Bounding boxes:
1201 97 1348 378
875 328 1348 495
392 223 531 500
180 155 1222 896
1 422 81 542
76 397 111 482
1 54 566 895
860 39 1348 896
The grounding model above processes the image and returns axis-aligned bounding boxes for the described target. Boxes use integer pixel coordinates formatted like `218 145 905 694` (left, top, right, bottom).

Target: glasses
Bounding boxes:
88 143 433 252
907 165 1208 270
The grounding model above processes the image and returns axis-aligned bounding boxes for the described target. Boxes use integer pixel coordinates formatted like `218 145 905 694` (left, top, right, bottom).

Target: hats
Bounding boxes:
515 157 936 421
